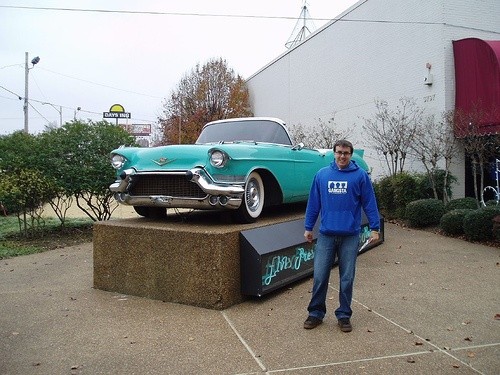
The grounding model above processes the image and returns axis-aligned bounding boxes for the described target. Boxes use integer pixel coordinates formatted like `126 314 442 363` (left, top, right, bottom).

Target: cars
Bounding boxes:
109 117 372 223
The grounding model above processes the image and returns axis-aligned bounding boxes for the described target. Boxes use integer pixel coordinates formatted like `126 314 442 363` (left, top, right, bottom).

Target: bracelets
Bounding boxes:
371 229 381 234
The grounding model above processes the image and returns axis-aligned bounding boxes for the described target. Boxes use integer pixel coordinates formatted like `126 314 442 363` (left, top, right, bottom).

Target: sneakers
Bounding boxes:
304 316 323 328
338 318 352 331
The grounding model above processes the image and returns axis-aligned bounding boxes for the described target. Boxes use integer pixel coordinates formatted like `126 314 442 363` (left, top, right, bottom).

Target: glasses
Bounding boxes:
335 151 352 155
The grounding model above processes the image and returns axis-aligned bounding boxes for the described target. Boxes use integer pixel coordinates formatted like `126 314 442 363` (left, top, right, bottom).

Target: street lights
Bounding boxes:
73 107 81 121
23 52 40 132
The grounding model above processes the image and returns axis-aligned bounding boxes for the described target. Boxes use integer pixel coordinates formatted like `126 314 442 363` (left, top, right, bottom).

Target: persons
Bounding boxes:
303 140 381 332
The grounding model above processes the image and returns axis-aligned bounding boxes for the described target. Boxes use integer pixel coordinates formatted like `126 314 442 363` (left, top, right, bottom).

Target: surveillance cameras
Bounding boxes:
31 57 40 64
